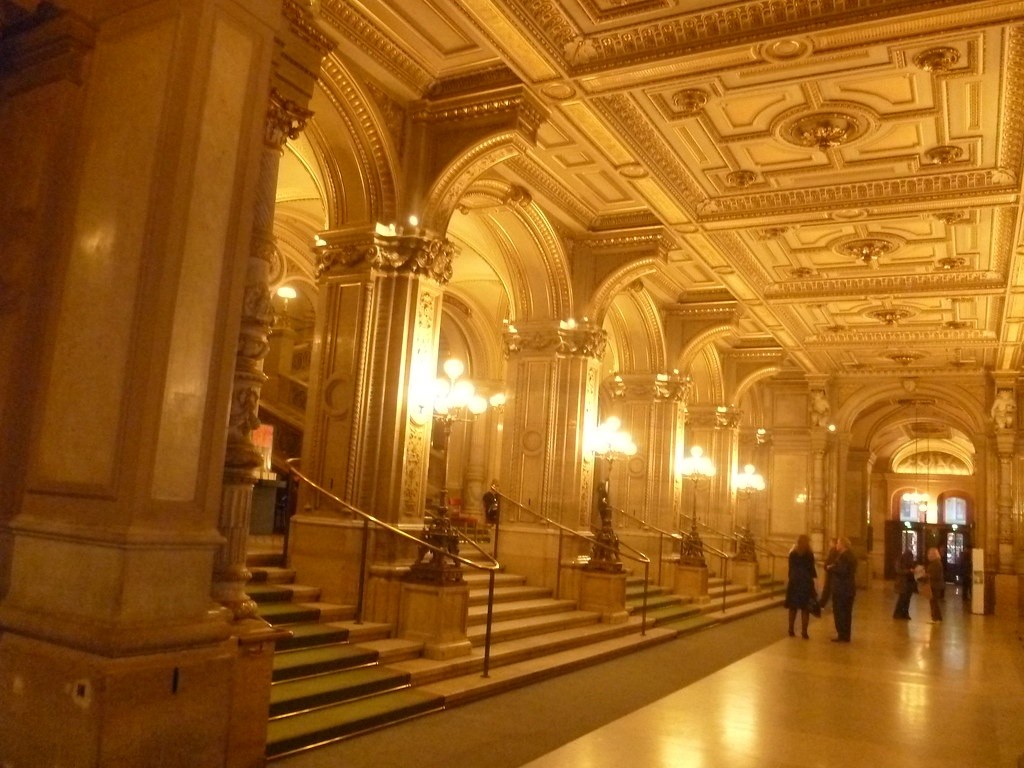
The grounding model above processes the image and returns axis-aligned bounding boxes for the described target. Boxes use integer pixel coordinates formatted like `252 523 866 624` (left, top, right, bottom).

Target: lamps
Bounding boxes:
489 393 505 414
903 399 930 503
277 286 296 318
919 402 939 513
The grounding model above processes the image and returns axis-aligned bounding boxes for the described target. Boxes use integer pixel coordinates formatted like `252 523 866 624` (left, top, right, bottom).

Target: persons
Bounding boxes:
818 536 856 642
959 545 972 608
892 547 943 624
783 534 821 640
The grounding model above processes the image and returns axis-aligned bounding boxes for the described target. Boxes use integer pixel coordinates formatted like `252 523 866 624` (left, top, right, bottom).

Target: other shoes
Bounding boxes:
893 614 911 620
788 629 795 637
830 637 851 643
926 620 943 625
801 631 809 640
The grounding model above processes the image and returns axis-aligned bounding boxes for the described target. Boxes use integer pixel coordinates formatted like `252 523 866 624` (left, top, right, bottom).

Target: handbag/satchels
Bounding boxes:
809 597 821 618
918 575 934 599
893 582 907 594
